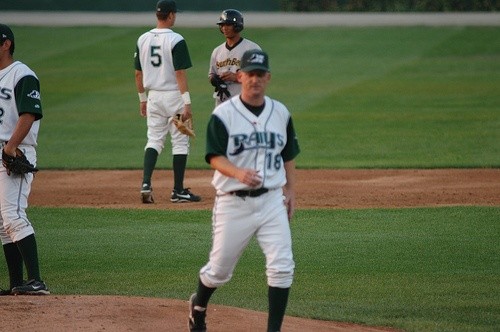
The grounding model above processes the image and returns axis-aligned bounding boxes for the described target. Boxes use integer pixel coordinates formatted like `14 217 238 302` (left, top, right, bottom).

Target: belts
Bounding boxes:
230 188 268 197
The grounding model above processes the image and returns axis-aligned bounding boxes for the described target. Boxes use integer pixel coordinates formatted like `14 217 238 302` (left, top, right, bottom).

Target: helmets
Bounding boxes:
216 9 243 33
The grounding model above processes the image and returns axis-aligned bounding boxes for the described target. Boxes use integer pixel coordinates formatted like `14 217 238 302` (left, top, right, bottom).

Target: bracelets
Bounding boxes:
138 90 147 103
181 91 191 106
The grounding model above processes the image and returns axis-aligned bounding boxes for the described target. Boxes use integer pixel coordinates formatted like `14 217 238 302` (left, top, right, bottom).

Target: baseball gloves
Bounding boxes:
172 113 196 138
2 146 40 176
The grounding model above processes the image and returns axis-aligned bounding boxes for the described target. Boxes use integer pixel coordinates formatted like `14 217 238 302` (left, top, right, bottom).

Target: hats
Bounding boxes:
240 50 270 72
156 0 183 12
0 24 14 40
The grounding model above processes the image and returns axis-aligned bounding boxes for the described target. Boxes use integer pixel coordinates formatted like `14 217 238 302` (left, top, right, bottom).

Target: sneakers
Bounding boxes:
141 183 154 203
12 279 50 295
170 188 201 202
188 293 207 332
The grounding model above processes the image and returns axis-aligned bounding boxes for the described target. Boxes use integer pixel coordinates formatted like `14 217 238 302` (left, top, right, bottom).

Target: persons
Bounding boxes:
207 9 263 108
133 0 201 203
0 22 51 295
188 49 301 332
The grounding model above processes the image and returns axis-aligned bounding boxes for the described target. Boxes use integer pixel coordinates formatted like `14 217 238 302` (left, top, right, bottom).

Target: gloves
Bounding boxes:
210 75 230 100
